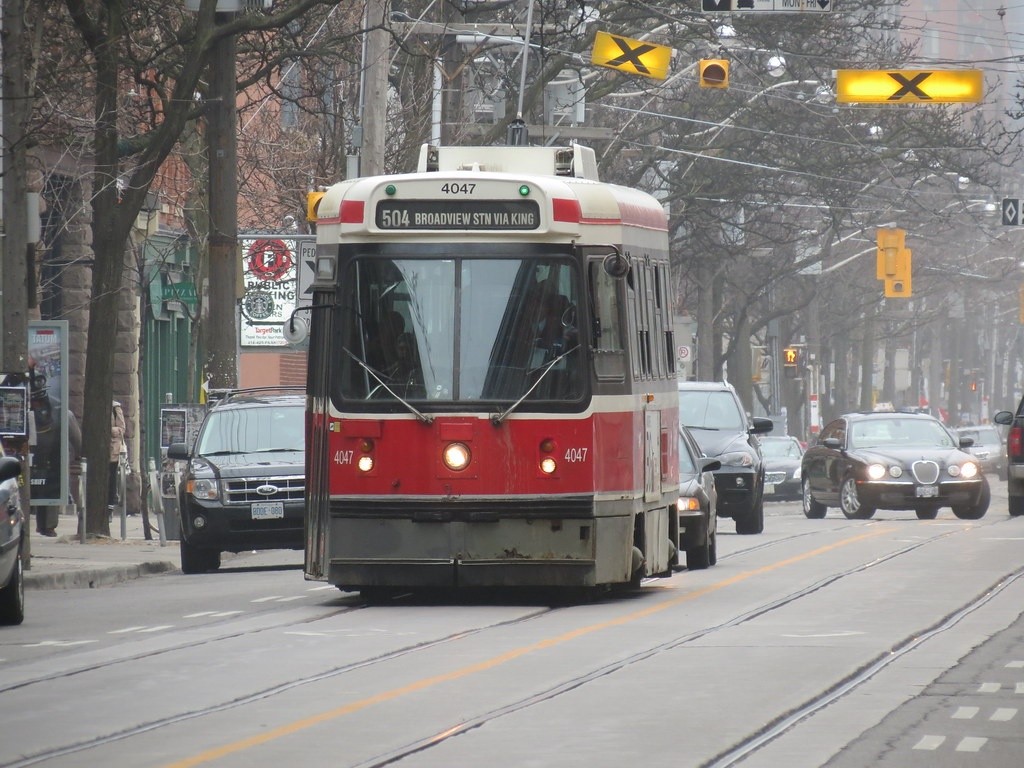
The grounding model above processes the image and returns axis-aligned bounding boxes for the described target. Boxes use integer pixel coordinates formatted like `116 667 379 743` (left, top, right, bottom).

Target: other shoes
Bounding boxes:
36 526 45 535
43 528 57 537
108 504 118 511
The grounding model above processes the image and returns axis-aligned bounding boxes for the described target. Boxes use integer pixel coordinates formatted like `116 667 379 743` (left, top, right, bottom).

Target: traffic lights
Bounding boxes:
783 349 798 367
699 59 729 88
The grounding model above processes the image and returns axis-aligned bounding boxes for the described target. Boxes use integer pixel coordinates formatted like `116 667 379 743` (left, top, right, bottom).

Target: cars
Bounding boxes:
994 395 1024 517
755 434 804 500
0 443 25 626
951 424 1010 482
678 418 723 571
801 412 992 520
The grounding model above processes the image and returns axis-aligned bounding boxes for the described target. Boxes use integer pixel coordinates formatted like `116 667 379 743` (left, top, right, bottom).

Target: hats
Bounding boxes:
397 332 415 344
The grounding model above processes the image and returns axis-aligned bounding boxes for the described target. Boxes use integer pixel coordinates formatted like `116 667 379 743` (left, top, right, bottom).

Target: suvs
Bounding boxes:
169 384 307 576
677 377 773 534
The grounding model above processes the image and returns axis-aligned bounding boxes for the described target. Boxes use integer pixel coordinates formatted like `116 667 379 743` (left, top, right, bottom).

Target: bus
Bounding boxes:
282 136 680 602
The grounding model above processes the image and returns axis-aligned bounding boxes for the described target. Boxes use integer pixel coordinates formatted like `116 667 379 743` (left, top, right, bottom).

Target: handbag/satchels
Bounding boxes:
117 428 131 476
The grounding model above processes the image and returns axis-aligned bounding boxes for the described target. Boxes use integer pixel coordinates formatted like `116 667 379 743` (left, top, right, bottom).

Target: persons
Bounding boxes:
370 311 405 367
36 409 82 537
107 400 126 522
514 293 562 348
381 333 416 379
